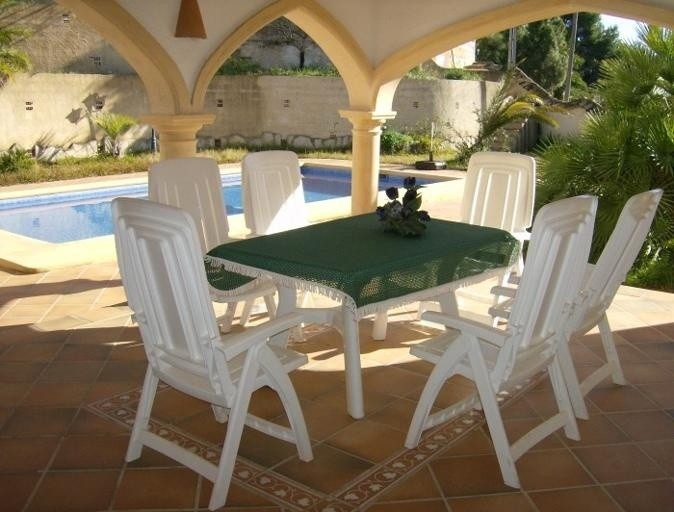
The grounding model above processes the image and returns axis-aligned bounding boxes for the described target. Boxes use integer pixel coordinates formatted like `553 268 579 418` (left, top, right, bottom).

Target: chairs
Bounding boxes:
111 196 316 510
403 193 600 492
507 187 663 422
418 150 537 329
147 156 276 334
242 149 308 236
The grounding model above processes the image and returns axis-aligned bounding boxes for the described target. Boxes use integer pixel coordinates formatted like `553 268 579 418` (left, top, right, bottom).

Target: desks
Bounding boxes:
203 208 521 420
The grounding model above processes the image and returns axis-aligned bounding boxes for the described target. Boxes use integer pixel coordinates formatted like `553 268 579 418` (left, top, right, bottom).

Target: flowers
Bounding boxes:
375 176 430 237
